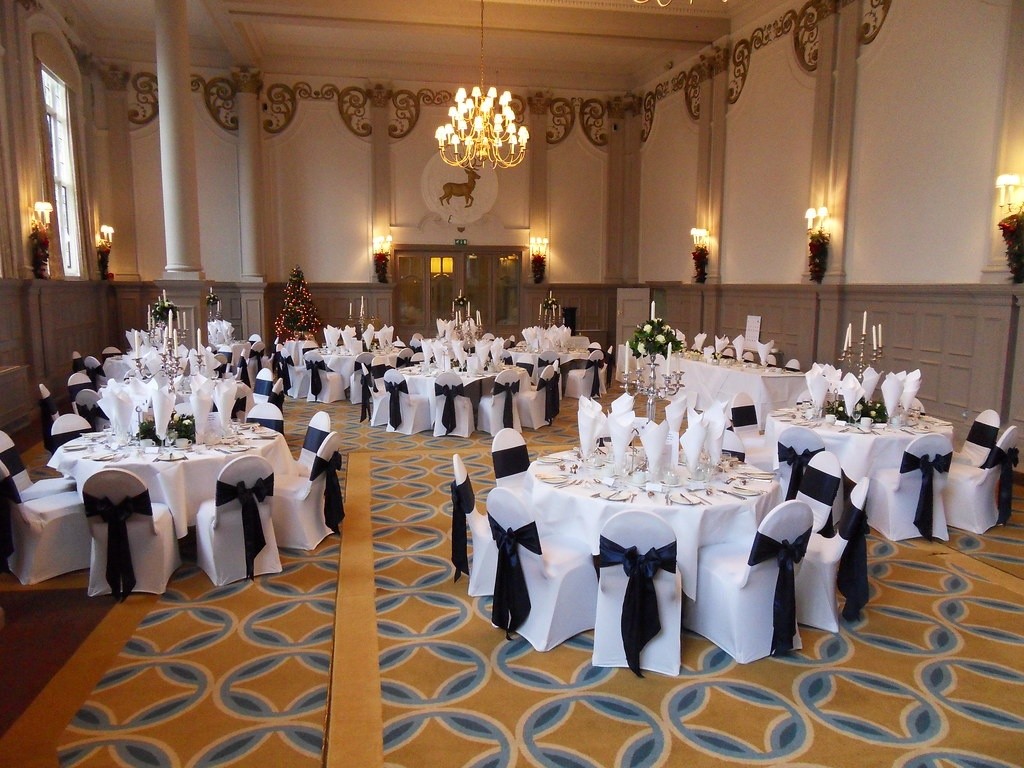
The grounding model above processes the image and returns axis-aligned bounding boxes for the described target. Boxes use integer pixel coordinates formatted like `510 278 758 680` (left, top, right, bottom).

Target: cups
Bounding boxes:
175 438 192 448
679 350 782 374
824 414 836 424
399 359 523 377
322 343 344 354
139 438 155 447
860 417 873 428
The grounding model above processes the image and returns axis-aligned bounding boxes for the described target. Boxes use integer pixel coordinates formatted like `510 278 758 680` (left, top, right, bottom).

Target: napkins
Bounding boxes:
669 328 776 367
323 317 572 372
805 361 923 417
98 319 241 441
577 390 732 474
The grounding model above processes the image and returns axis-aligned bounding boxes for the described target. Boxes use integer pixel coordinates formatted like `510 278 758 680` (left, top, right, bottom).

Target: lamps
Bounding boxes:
0 0 1024 284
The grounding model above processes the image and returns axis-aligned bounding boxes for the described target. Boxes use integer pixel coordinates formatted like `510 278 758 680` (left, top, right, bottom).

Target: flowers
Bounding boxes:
827 398 890 424
203 293 219 305
626 318 683 356
150 299 180 320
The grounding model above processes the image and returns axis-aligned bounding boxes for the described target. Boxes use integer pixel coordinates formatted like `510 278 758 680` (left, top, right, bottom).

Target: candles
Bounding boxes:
131 286 222 358
843 308 883 350
347 291 567 327
626 300 683 377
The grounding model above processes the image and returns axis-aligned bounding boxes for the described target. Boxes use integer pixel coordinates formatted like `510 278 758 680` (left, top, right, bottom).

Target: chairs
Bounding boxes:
0 345 175 599
730 390 763 445
351 332 613 437
196 334 347 588
777 425 824 488
944 408 1020 533
680 345 803 373
868 434 953 542
445 453 870 677
490 428 531 478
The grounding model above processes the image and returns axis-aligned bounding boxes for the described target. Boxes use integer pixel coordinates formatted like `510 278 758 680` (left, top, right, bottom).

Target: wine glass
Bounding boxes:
213 371 219 383
229 418 241 436
579 434 732 497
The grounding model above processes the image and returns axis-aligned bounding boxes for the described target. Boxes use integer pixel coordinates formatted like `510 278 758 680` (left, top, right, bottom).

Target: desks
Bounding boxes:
423 336 498 355
615 345 807 412
87 379 253 425
103 351 223 376
304 345 399 389
500 447 781 571
508 347 591 378
47 423 295 541
395 366 527 426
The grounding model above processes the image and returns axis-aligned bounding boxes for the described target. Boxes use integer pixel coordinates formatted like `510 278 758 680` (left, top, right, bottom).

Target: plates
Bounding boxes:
66 422 279 462
773 403 933 436
534 446 773 505
